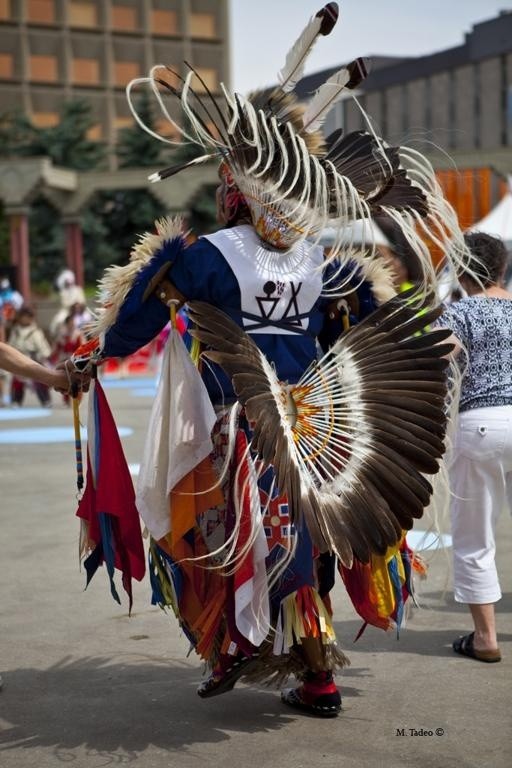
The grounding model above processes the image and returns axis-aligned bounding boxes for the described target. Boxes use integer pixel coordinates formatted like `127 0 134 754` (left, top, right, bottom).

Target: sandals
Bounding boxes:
451 629 502 663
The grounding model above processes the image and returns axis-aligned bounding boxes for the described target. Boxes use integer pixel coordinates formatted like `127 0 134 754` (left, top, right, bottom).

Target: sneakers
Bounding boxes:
278 686 344 718
194 636 273 700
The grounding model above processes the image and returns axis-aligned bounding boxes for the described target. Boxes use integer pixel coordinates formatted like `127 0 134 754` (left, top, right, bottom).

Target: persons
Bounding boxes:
47 85 401 719
427 227 511 663
0 266 104 410
